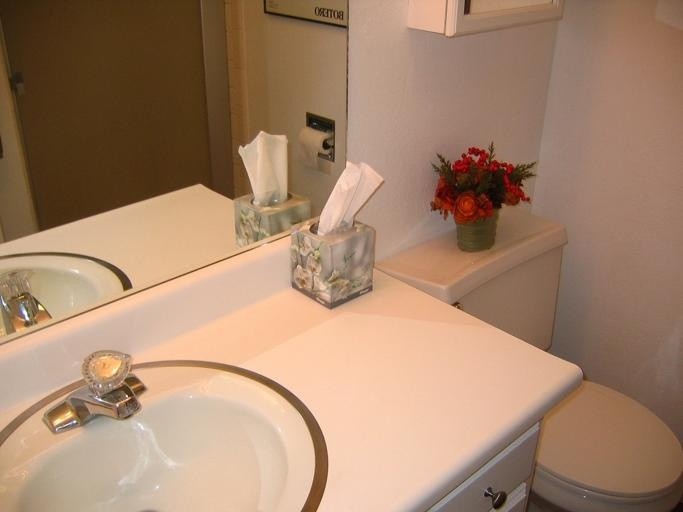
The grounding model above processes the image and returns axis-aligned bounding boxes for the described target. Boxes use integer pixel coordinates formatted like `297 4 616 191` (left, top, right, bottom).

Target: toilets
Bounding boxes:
374 210 683 512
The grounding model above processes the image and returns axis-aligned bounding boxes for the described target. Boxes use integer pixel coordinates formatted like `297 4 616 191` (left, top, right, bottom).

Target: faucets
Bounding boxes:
0 269 52 336
66 351 141 426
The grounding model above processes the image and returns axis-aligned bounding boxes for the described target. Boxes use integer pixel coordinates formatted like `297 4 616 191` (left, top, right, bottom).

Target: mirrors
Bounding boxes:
0 0 347 346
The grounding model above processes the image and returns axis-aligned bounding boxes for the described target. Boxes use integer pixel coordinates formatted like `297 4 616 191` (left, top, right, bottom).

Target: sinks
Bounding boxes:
0 362 329 512
0 252 133 346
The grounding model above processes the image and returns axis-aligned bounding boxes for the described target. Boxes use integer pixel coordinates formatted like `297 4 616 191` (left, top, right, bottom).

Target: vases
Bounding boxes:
454 211 502 251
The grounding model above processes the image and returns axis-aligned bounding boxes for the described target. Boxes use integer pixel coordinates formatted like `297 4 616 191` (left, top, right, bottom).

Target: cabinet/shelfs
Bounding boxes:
422 417 543 511
402 0 566 41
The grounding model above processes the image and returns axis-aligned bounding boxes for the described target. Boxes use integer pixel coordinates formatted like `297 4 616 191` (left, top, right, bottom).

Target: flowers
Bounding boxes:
427 139 540 225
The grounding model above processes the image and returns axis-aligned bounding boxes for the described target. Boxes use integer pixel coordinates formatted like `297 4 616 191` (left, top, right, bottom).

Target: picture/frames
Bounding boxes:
261 0 346 29
